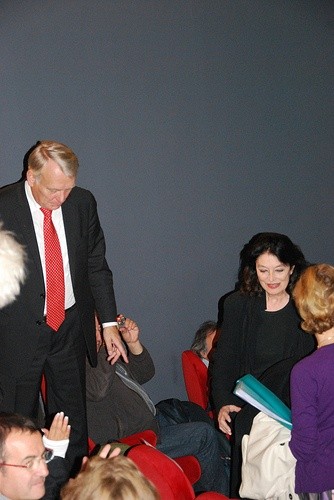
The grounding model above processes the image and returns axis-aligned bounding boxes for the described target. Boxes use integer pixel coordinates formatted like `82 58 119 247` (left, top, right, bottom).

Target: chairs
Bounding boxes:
87 429 233 500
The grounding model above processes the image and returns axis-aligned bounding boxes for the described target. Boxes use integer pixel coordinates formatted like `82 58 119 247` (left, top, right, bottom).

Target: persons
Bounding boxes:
86 311 232 498
0 221 27 309
238 264 334 500
191 321 217 369
0 413 121 500
60 456 159 500
207 231 318 500
0 140 129 480
41 412 70 500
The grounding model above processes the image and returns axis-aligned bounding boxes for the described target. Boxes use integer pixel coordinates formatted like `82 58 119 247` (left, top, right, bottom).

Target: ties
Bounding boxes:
39 204 66 332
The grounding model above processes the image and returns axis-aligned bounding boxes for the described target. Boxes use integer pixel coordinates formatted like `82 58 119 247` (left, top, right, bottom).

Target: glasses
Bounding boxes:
1 448 55 473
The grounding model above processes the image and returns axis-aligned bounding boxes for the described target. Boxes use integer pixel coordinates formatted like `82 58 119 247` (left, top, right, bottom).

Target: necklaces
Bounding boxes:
317 336 334 347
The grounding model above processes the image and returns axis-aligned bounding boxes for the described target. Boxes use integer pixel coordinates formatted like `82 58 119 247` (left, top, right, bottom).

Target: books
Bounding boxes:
233 374 293 431
